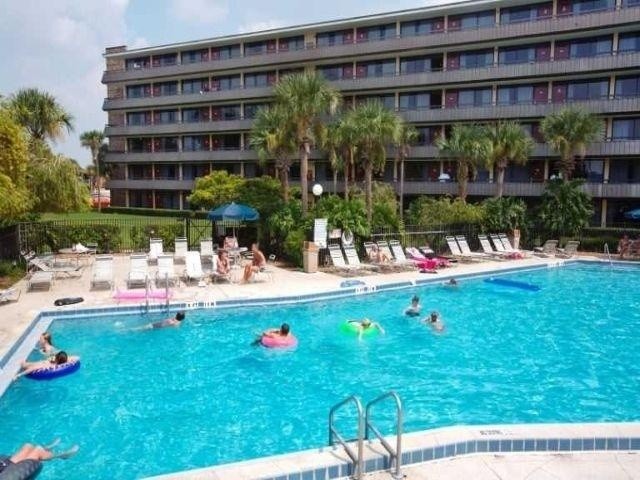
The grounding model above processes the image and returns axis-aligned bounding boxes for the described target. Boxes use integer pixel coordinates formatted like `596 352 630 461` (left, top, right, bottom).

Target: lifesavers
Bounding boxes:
341 319 379 338
23 354 80 379
262 331 298 348
0 454 42 480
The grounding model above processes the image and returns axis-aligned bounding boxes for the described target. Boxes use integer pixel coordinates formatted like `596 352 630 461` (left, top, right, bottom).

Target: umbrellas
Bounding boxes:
206 201 260 238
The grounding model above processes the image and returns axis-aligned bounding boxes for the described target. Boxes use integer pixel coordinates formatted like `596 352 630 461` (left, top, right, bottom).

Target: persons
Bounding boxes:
401 294 423 317
445 277 459 287
212 235 266 285
368 245 394 265
119 310 186 331
617 233 632 259
420 310 441 323
38 332 53 355
12 350 82 382
347 317 386 342
261 322 291 340
0 434 81 475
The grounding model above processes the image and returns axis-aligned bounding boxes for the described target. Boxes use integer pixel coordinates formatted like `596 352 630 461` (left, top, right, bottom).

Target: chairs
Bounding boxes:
1 236 278 302
324 231 580 276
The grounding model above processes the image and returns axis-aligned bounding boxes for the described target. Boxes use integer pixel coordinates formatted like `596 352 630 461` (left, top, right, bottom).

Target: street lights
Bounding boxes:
311 183 324 203
